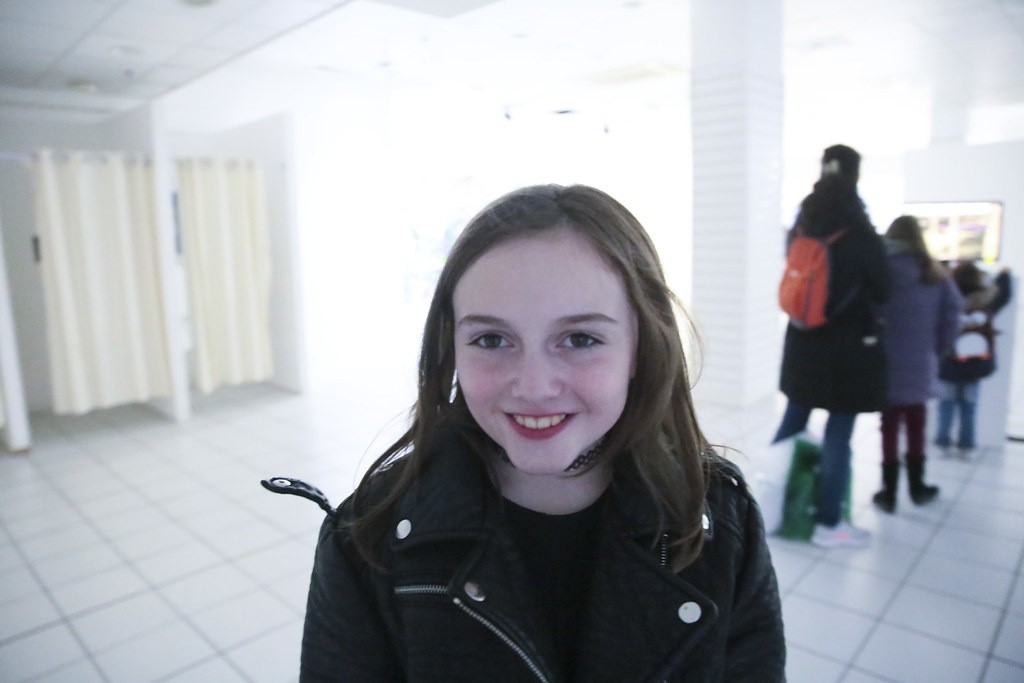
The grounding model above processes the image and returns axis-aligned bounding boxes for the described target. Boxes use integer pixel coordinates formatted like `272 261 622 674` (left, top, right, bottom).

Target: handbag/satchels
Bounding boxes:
771 441 851 540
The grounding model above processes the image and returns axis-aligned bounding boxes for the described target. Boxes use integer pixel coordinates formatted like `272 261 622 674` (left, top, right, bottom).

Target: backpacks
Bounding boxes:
778 221 875 326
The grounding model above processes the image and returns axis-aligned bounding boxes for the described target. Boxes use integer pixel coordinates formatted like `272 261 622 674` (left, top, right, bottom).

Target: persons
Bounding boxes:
773 144 892 547
872 214 962 515
934 259 1012 448
260 186 788 682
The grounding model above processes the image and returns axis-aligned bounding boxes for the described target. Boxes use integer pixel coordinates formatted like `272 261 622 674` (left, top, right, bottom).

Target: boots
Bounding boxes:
873 460 899 512
905 453 938 506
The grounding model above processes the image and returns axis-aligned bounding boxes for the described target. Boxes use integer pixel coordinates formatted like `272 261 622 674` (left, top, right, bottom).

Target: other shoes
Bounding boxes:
810 518 872 548
